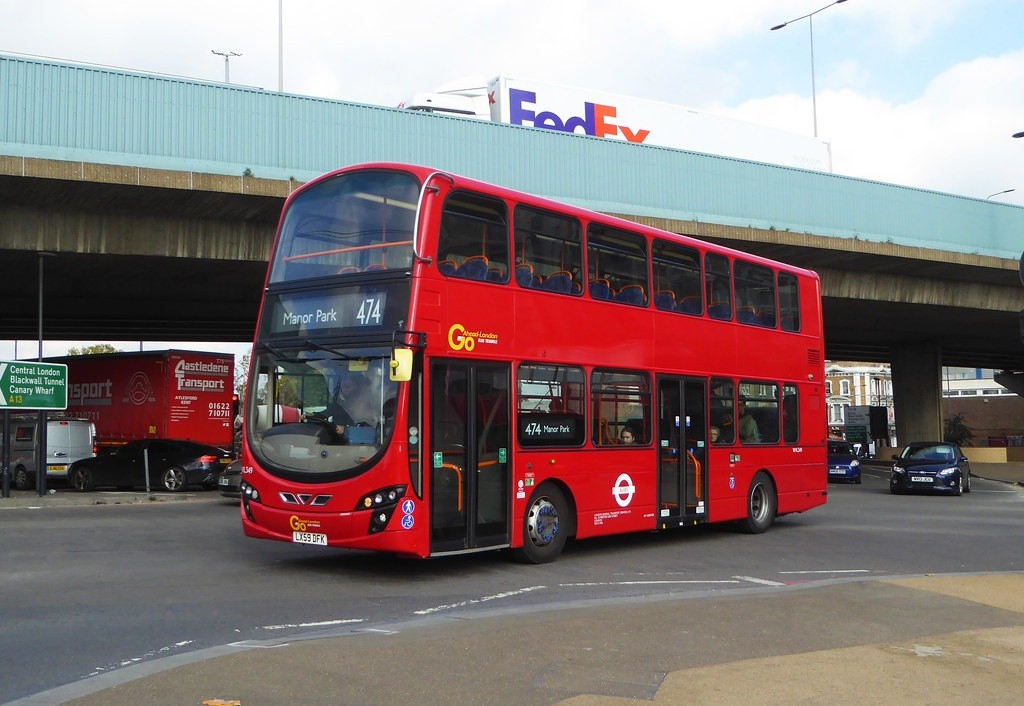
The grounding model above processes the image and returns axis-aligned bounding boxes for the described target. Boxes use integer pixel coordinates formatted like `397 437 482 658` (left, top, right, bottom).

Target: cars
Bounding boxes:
889 442 972 496
67 438 235 492
219 459 241 498
828 440 862 484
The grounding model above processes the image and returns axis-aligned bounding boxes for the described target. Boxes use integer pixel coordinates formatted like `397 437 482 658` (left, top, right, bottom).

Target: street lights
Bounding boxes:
854 385 866 406
873 378 880 406
770 0 848 138
986 189 1014 200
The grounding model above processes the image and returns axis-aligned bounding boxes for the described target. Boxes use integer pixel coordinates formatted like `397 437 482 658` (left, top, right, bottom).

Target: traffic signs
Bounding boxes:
0 361 68 410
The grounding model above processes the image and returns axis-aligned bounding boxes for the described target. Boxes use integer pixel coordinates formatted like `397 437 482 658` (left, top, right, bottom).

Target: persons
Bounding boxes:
620 426 637 445
697 383 761 444
299 373 383 444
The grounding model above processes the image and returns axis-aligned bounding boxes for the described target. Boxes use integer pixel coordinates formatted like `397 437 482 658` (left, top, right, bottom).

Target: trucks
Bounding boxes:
853 441 875 459
0 412 98 491
395 76 705 155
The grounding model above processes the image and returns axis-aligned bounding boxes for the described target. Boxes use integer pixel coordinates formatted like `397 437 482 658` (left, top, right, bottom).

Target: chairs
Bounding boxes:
337 264 389 274
439 255 795 331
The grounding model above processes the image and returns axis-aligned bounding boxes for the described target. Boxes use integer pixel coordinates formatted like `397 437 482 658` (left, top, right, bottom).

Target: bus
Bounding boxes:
239 162 828 563
